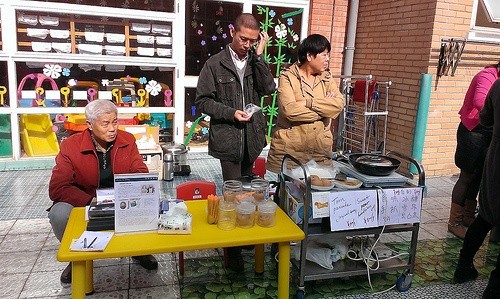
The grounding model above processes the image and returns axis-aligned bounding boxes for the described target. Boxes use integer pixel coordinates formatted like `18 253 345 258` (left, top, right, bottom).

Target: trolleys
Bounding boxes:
272 149 425 298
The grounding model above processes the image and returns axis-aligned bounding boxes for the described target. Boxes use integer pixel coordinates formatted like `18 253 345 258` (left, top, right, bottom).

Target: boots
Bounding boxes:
447 199 477 239
481 255 500 299
452 214 495 284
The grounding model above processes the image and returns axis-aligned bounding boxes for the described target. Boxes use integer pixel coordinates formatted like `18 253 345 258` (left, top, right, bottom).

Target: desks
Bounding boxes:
56 196 306 299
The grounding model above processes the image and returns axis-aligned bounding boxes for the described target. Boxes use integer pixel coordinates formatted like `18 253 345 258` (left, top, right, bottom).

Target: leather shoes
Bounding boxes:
60 261 71 283
132 255 158 270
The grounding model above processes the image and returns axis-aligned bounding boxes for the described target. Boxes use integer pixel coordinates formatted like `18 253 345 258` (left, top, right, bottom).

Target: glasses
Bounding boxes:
235 31 259 43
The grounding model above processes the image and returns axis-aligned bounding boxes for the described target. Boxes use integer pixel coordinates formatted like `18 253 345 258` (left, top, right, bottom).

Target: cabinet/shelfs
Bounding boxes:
280 150 426 299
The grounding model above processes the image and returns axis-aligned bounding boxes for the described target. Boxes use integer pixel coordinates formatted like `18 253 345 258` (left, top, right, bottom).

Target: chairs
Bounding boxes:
176 180 230 276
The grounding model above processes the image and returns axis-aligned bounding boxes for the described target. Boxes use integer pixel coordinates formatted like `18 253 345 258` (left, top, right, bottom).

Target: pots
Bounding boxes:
348 141 402 176
159 141 191 165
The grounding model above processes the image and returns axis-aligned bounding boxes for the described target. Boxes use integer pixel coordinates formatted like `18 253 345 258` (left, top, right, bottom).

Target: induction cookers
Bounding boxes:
346 166 409 188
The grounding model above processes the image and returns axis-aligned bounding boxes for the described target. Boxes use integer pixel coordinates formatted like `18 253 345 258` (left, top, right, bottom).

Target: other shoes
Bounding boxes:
223 245 254 272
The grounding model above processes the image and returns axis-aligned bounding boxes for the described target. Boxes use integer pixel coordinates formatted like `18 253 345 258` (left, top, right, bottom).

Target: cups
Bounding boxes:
250 179 269 205
207 197 219 225
215 201 238 230
257 201 277 228
236 202 256 229
222 180 243 204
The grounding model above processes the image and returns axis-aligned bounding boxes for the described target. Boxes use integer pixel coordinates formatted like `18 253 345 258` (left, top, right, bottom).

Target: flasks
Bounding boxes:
163 152 173 181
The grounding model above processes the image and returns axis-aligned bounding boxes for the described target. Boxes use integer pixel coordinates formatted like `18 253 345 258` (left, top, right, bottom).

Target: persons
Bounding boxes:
448 59 500 239
451 68 500 299
196 13 277 274
264 34 346 260
45 99 159 285
